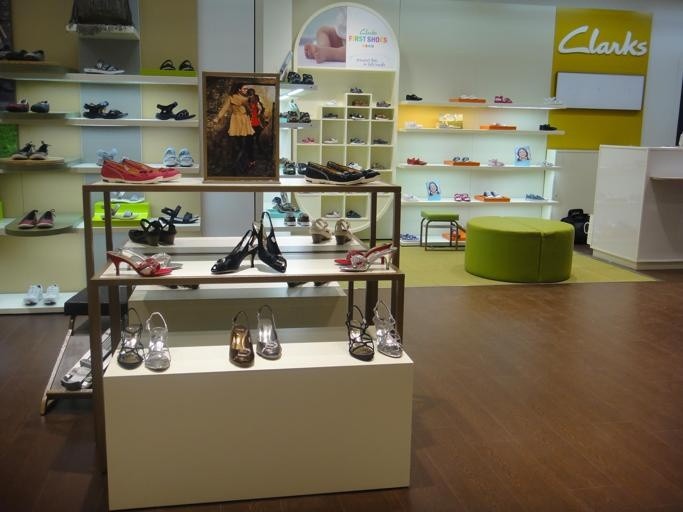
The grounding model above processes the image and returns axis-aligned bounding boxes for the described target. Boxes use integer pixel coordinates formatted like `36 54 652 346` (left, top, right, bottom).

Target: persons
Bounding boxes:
303 24 346 64
428 181 440 196
517 147 530 161
212 82 264 177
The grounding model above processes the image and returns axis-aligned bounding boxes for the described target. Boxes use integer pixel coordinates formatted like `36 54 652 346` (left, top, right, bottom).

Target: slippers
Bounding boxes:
83 61 124 75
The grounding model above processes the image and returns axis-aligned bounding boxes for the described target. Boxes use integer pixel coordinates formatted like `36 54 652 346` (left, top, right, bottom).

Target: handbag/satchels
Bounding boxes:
561 208 593 244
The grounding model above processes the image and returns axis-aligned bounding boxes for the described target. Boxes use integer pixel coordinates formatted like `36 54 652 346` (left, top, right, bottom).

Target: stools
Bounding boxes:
416 210 459 252
462 215 575 284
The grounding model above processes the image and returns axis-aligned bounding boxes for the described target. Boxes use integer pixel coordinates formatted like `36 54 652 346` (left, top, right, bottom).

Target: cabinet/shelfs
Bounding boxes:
395 99 566 249
289 67 400 235
83 178 415 510
539 145 598 231
0 58 206 315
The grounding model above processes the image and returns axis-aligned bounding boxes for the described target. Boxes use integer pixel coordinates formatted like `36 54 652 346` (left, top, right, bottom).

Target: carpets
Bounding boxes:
334 245 665 291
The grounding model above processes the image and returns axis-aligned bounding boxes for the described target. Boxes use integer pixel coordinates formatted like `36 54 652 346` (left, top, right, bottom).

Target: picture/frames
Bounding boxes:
200 71 282 184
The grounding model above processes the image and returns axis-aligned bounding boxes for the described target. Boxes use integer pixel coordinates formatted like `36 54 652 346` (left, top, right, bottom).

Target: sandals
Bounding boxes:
83 101 128 119
110 192 144 203
94 147 193 183
100 204 138 220
160 205 199 223
179 60 193 71
160 60 176 70
155 101 195 120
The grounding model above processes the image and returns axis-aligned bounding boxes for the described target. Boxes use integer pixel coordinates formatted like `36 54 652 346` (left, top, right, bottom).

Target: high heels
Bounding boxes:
344 304 374 361
334 219 352 244
249 212 286 273
371 300 403 358
210 230 259 275
229 311 254 367
333 242 397 272
255 304 282 360
310 217 331 244
144 311 170 370
128 217 176 246
116 307 143 366
105 248 182 276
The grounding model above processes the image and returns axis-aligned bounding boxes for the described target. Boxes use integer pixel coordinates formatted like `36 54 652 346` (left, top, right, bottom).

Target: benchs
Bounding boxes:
39 278 134 419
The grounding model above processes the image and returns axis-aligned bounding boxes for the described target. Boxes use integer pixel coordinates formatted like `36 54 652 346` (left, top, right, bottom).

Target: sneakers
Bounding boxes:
16 208 56 229
282 72 391 185
5 50 44 61
30 101 49 112
11 139 53 160
324 210 341 218
296 212 309 226
346 209 361 218
22 283 60 305
282 214 296 226
4 101 28 113
400 94 564 242
